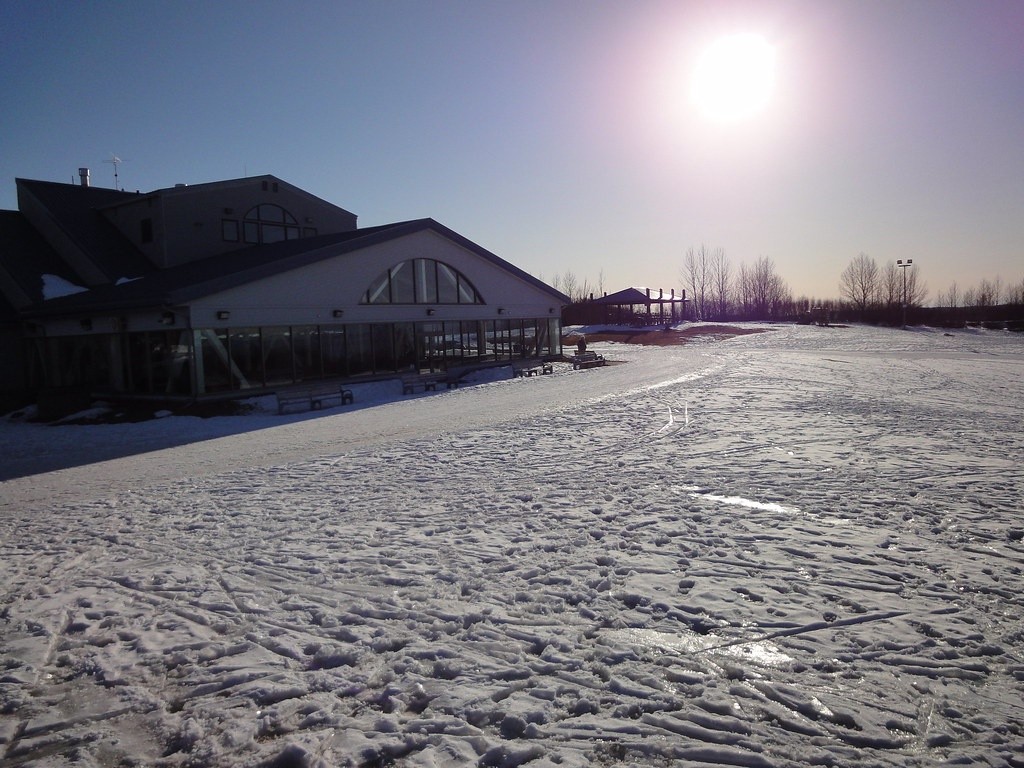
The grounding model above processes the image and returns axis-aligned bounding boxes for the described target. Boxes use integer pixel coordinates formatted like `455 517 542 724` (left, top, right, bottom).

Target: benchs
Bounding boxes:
401 371 459 395
571 350 603 370
512 359 553 378
493 349 513 355
275 384 355 416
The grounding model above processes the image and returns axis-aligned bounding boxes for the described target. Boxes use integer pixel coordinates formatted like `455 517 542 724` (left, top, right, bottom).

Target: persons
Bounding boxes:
578 336 587 351
664 322 670 333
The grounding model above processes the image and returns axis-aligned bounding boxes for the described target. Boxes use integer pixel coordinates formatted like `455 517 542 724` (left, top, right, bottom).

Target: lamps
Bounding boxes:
217 311 230 319
157 311 176 326
79 318 92 330
550 308 556 313
224 208 233 215
427 309 436 316
498 309 504 314
25 322 46 336
306 217 314 223
334 310 345 317
